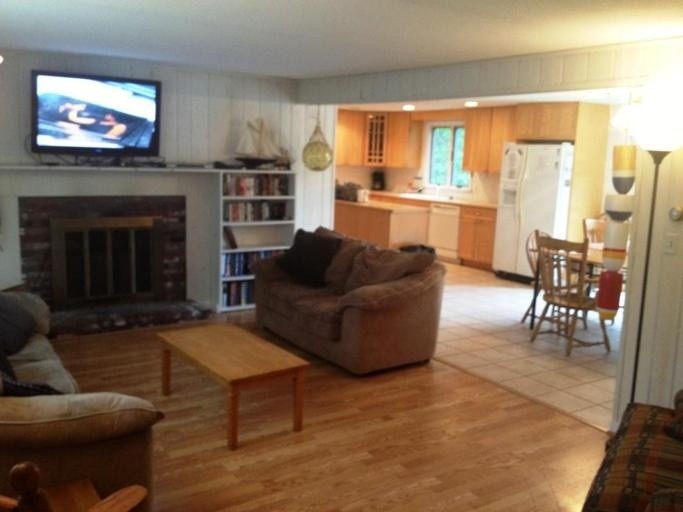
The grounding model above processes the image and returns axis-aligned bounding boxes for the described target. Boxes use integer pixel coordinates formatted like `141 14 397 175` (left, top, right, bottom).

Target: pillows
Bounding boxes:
272 227 437 293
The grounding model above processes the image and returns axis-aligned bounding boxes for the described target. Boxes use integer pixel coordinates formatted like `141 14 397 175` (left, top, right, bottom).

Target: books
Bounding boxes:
220 173 294 310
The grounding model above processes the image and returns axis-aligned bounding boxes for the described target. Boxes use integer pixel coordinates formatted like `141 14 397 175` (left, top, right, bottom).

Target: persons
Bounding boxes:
48 95 74 120
87 111 120 137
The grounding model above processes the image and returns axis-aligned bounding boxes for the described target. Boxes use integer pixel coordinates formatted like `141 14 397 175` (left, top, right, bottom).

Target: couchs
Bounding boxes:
249 225 447 379
0 284 164 509
578 384 683 511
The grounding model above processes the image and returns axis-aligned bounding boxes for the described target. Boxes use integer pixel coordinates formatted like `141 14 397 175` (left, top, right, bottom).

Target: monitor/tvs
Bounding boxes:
30 69 161 157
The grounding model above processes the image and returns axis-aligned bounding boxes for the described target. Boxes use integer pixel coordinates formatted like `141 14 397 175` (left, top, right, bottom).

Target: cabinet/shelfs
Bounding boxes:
363 113 419 168
459 107 512 174
333 107 360 166
510 102 612 144
190 172 298 310
456 207 497 268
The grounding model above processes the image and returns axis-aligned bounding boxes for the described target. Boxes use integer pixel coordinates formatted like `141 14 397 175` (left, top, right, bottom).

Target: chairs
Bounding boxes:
520 217 628 361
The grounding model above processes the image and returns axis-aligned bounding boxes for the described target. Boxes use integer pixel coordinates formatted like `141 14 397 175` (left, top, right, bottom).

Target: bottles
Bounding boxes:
412 176 422 189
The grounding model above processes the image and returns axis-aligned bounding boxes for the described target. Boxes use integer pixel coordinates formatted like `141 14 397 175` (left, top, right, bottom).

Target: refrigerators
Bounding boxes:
492 141 575 284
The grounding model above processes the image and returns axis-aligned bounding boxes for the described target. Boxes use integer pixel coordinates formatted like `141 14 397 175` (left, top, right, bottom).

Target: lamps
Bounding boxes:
300 87 334 171
614 120 682 401
